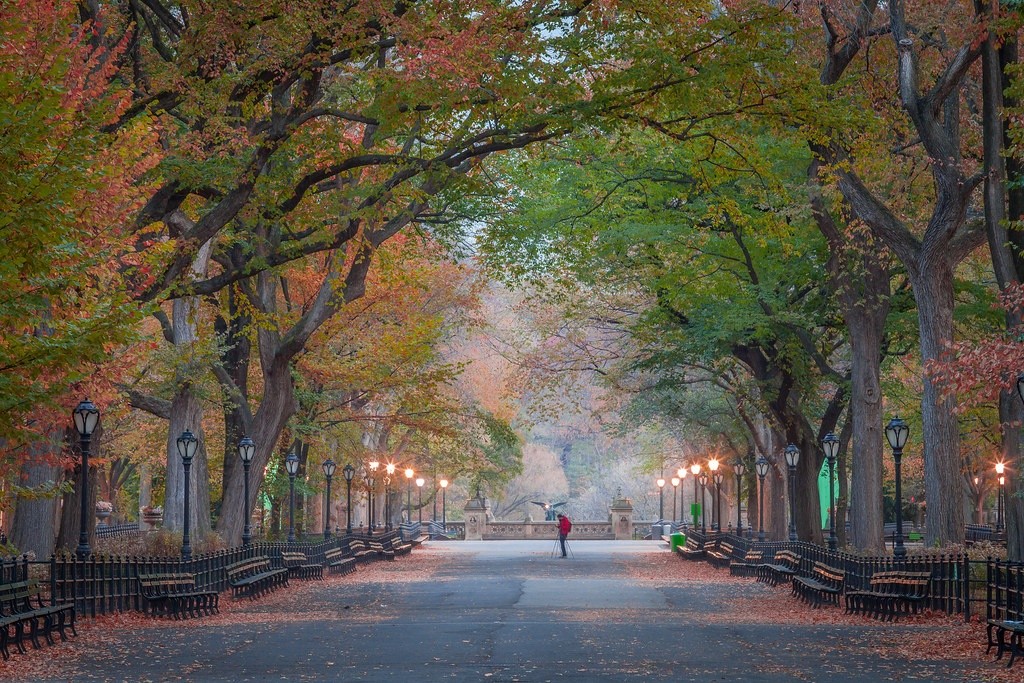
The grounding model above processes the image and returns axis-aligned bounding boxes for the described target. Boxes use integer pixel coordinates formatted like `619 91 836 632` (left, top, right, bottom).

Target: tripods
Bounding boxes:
551 529 574 560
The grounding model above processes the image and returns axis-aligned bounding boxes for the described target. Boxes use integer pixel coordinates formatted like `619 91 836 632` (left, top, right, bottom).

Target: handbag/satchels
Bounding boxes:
568 522 571 532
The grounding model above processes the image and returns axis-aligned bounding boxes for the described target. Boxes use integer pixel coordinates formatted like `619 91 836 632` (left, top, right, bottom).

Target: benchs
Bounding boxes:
791 560 845 609
756 549 802 587
730 550 764 576
369 542 395 562
845 572 932 623
985 587 1024 670
282 551 324 581
138 572 219 622
225 555 290 603
0 577 79 660
391 536 411 557
349 540 378 565
324 547 357 577
707 541 734 569
677 537 700 560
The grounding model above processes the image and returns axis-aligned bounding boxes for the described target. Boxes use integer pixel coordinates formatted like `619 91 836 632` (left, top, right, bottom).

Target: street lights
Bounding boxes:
714 470 724 534
678 468 687 522
657 479 666 520
884 415 910 556
708 459 719 530
285 452 299 541
691 463 701 532
820 431 841 552
754 455 769 541
237 436 256 546
342 464 355 529
732 459 745 538
698 472 709 535
784 442 802 541
71 396 101 559
671 477 680 521
995 462 1005 533
363 460 448 535
322 461 337 540
176 429 198 559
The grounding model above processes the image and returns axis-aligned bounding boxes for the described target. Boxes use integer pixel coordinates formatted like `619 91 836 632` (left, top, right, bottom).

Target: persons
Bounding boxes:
555 514 569 559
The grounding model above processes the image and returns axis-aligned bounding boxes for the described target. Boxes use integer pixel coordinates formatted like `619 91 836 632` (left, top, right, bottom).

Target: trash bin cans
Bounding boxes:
652 525 662 540
670 532 685 552
